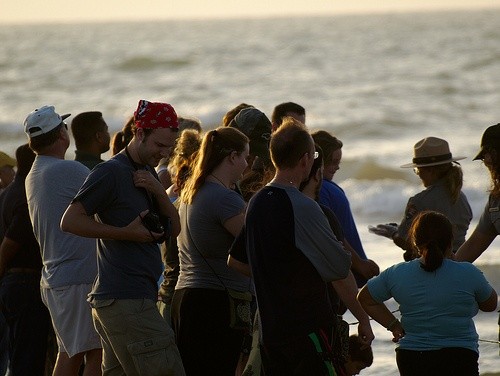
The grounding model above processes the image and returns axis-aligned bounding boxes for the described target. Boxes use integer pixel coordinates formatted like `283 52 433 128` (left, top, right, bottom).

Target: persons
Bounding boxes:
450 122 500 376
369 137 473 261
0 98 380 376
358 212 497 376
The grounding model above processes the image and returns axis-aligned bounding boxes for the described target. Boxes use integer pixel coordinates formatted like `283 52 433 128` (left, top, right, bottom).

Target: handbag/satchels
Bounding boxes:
228 287 253 331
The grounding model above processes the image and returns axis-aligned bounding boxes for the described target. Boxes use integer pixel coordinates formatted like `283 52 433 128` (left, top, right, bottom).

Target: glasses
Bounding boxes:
58 121 68 131
413 165 435 175
298 150 319 159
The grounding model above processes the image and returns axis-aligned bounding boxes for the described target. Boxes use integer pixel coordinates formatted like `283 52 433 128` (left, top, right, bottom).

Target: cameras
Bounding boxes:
141 208 170 242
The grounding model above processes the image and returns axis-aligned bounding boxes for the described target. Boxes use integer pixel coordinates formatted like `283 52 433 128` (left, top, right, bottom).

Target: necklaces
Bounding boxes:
209 173 227 187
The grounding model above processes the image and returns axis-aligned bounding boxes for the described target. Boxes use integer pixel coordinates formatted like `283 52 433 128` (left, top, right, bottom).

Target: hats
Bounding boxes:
473 122 500 160
0 151 16 169
234 107 272 158
24 105 71 137
400 137 467 168
